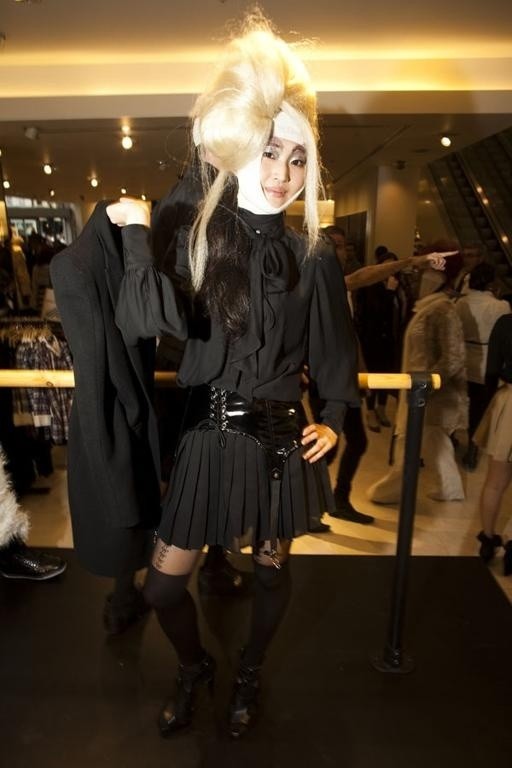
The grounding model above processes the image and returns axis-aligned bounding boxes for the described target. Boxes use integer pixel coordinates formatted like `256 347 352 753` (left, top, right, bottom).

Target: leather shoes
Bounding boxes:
0 537 68 580
308 523 330 532
327 501 374 523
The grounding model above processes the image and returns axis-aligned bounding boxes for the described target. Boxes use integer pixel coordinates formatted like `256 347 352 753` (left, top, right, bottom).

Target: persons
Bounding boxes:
105 6 359 737
359 226 511 504
0 449 66 580
470 312 511 577
307 227 458 533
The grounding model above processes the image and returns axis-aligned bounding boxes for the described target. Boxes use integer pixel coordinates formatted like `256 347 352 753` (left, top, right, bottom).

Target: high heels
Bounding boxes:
504 541 512 577
227 654 266 738
158 652 218 738
476 531 503 562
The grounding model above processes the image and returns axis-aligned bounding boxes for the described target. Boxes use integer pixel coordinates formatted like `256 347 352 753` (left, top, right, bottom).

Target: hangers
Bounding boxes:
0 317 55 344
